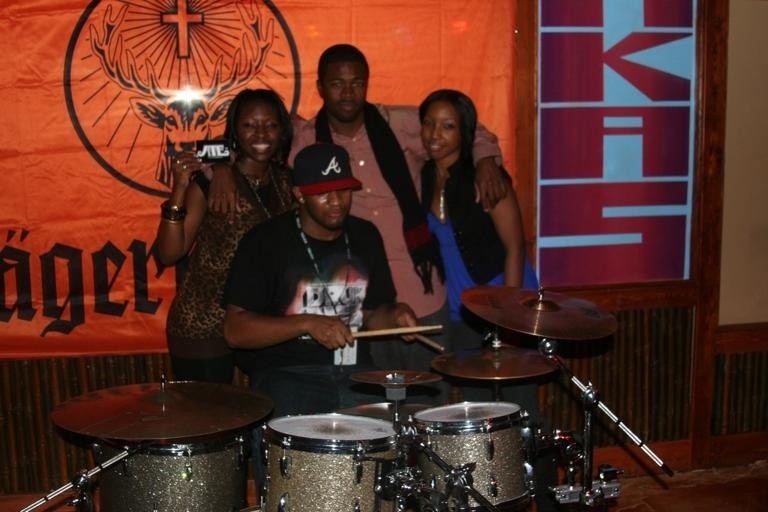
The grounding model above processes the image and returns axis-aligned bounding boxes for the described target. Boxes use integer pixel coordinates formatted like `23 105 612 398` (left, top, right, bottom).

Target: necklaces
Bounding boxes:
435 166 449 221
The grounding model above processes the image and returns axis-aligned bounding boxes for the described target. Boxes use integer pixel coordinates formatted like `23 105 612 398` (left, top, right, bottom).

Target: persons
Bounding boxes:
156 88 301 386
206 44 507 407
222 145 449 417
415 90 536 353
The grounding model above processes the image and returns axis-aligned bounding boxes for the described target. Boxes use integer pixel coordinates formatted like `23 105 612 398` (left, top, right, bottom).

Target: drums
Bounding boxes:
413 401 532 511
91 434 251 511
256 412 408 512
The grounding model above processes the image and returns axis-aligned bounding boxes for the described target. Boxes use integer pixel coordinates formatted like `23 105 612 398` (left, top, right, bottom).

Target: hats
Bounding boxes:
295 141 361 194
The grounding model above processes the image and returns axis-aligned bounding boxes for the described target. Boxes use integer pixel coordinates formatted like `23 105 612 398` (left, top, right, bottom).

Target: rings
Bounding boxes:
176 159 183 166
180 163 187 170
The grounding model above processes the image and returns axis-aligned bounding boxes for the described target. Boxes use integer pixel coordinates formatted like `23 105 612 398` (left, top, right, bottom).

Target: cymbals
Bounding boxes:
461 285 618 341
431 345 559 380
52 378 274 439
349 370 442 389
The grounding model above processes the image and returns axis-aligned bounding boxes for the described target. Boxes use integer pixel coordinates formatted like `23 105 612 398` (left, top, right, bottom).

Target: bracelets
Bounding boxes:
159 199 187 224
